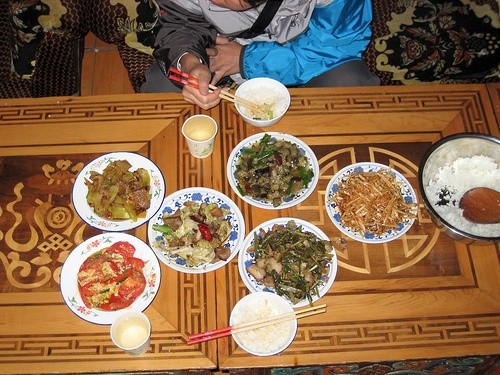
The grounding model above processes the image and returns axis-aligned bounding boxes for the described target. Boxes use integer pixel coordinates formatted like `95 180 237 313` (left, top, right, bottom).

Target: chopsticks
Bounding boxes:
186 305 327 346
168 66 262 114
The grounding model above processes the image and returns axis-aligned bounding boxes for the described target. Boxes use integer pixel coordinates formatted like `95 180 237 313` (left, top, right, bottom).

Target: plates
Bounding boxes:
325 162 418 245
148 187 246 273
72 151 167 232
59 231 162 325
226 131 319 210
238 217 338 307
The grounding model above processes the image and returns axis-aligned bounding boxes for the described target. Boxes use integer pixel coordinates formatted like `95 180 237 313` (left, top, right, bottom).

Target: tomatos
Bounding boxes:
76 241 147 311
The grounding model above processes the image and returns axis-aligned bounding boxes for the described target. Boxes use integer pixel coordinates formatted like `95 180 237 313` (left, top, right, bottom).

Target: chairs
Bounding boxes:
0 0 500 98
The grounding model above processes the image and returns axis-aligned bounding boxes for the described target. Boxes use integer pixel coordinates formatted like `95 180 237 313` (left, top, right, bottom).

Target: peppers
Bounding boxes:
198 222 213 241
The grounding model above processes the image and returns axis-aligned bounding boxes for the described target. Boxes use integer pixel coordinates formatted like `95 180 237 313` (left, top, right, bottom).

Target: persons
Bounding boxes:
140 0 380 111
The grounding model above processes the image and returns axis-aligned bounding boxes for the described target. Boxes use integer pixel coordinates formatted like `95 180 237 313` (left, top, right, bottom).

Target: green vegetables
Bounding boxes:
254 224 334 308
152 225 172 235
234 134 314 198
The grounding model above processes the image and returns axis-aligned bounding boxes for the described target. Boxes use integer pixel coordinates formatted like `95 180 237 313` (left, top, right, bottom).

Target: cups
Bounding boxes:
181 114 218 158
110 310 151 355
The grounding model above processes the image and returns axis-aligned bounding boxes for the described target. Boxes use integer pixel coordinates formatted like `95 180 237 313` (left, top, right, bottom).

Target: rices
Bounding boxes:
425 154 500 238
238 88 283 121
234 307 291 352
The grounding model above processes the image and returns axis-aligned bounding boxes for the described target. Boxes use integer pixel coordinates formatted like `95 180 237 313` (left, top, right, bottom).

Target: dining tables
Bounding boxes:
1 83 500 375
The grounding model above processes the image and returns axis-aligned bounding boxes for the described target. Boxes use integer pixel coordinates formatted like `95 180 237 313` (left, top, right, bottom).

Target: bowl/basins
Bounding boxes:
229 293 298 357
233 77 291 129
418 133 500 241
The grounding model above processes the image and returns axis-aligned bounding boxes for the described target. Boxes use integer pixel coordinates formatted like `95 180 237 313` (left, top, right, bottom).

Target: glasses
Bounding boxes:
207 0 267 12
